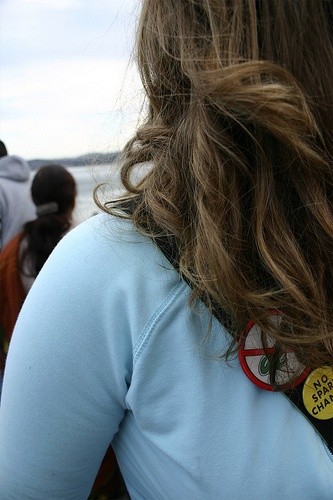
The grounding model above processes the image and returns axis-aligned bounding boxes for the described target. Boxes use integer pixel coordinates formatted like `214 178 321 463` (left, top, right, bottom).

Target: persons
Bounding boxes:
0 165 77 366
0 0 333 500
0 141 37 243
